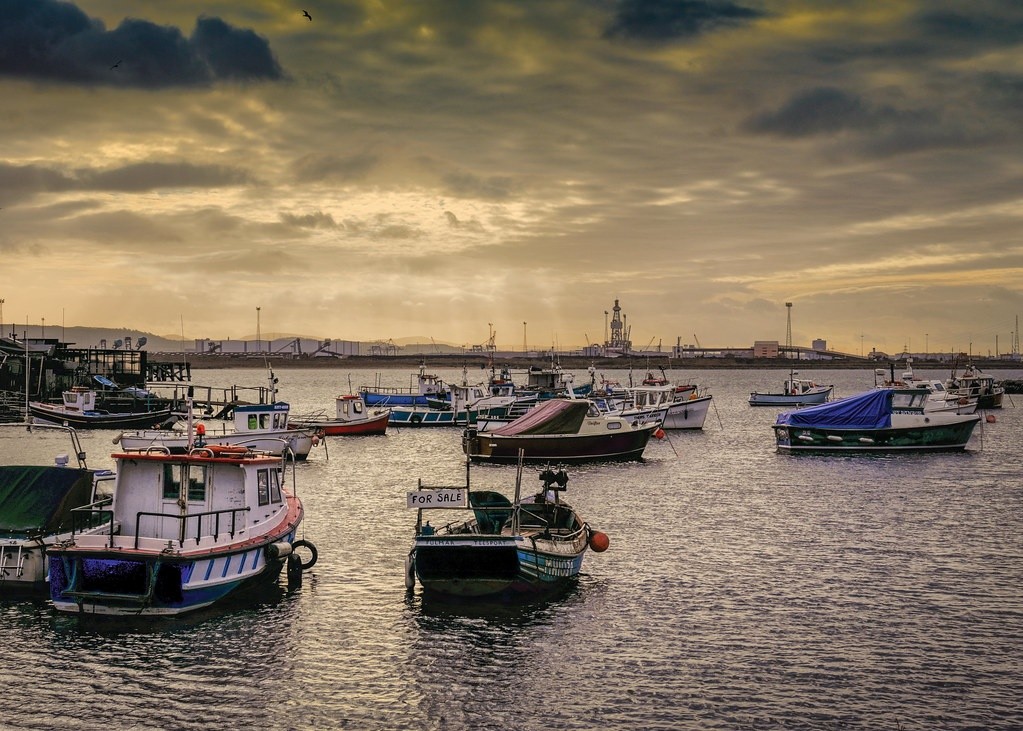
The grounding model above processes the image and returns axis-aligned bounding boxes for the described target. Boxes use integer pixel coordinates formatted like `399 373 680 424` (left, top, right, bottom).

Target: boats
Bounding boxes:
873 347 1006 413
0 357 320 585
284 389 394 438
350 345 712 433
404 404 592 608
773 382 985 459
457 392 663 464
42 396 306 624
749 357 836 408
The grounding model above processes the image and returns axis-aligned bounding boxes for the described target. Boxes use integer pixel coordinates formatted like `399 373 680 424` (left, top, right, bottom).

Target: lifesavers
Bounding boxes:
291 541 318 569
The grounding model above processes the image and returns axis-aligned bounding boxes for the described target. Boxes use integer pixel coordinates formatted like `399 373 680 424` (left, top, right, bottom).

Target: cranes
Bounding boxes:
487 297 700 356
